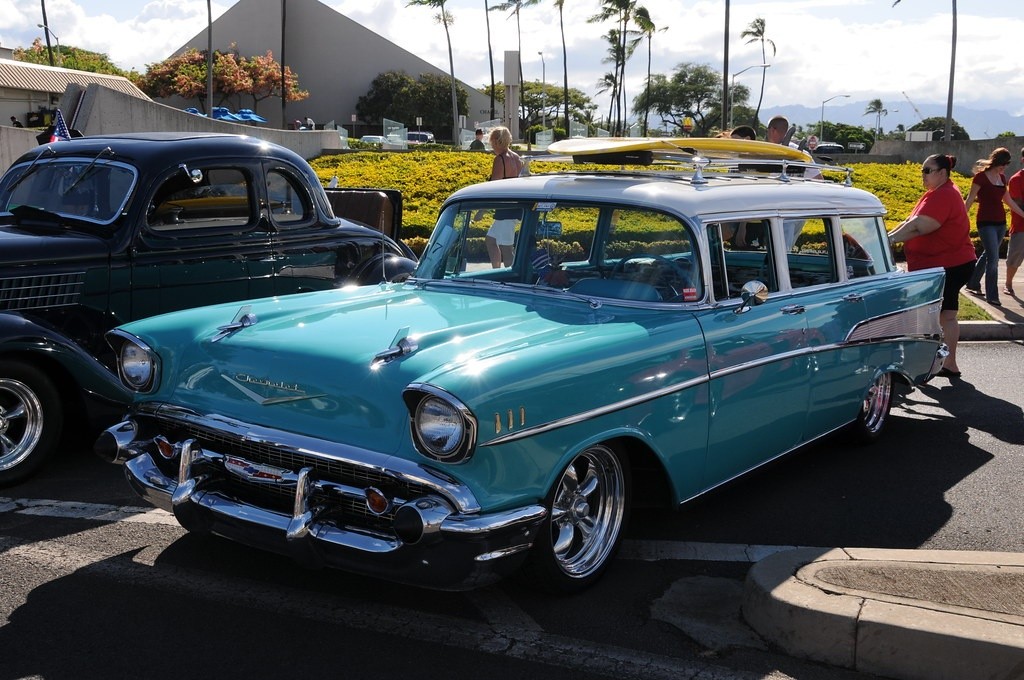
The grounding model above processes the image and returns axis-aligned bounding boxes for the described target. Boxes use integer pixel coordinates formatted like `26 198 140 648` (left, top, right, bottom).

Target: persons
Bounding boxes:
304 115 315 129
966 150 1024 306
141 166 241 219
466 129 485 150
10 116 23 128
294 119 301 130
887 153 978 378
1003 146 1024 306
718 115 824 254
485 126 525 269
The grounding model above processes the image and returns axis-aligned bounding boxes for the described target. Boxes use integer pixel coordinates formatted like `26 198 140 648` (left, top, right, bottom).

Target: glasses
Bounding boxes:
1000 161 1011 166
921 167 943 175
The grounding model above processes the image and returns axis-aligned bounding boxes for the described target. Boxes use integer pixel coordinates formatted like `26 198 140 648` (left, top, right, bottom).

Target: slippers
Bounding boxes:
965 289 1001 306
940 366 962 375
1003 286 1014 295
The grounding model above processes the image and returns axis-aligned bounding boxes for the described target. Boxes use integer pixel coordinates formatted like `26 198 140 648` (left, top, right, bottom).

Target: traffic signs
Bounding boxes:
848 142 866 150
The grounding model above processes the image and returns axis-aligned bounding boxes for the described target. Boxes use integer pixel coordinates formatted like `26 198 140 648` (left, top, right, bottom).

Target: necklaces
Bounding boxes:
987 172 1001 186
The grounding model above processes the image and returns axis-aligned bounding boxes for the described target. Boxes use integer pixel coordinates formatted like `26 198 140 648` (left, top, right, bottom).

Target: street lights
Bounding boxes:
538 52 546 145
875 110 899 142
820 95 850 144
730 64 770 129
38 24 60 66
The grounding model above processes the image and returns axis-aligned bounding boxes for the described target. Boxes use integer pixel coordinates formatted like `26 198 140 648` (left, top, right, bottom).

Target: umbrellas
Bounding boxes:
184 107 266 126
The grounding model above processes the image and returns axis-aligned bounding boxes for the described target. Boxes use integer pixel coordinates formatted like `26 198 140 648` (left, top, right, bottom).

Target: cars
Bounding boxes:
360 135 404 149
392 132 436 145
101 136 951 601
0 131 420 491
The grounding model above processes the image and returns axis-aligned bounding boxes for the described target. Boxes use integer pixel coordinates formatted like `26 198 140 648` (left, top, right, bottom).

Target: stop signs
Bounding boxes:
807 136 819 149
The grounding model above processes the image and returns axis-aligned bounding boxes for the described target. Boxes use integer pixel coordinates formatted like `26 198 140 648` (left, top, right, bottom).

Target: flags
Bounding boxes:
51 112 71 142
